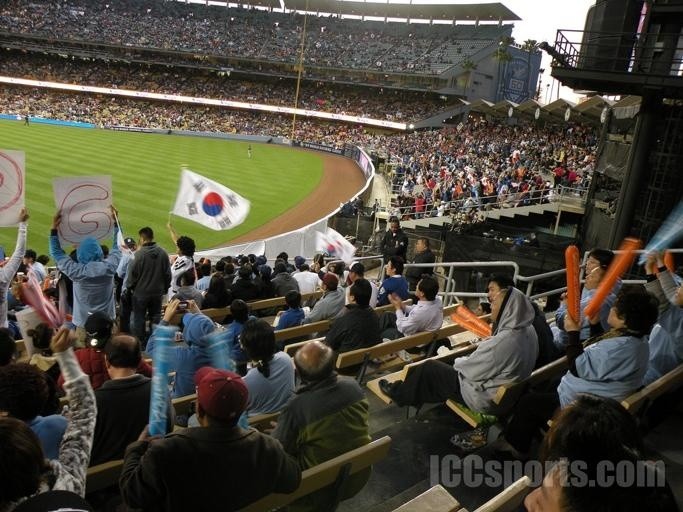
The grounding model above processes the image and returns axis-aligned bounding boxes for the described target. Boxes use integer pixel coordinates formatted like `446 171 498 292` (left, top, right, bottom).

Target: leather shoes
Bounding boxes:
379 379 405 408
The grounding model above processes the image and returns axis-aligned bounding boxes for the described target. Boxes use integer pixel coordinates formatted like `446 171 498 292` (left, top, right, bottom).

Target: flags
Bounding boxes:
169 166 252 233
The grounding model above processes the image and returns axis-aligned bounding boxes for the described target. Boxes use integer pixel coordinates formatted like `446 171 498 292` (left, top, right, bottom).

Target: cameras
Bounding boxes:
177 302 188 314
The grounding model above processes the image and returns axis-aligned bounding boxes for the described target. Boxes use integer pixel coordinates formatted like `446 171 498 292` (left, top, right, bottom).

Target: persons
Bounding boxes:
367 118 681 510
0 2 462 148
0 205 380 509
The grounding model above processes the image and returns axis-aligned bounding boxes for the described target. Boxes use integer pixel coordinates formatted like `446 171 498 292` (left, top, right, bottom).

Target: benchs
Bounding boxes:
339 24 498 75
15 290 683 512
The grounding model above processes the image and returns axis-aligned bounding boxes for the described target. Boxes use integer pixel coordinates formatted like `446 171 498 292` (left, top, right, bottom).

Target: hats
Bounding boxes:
194 367 248 419
85 311 114 348
125 238 136 245
344 261 364 272
318 270 338 288
183 313 195 326
295 256 305 266
